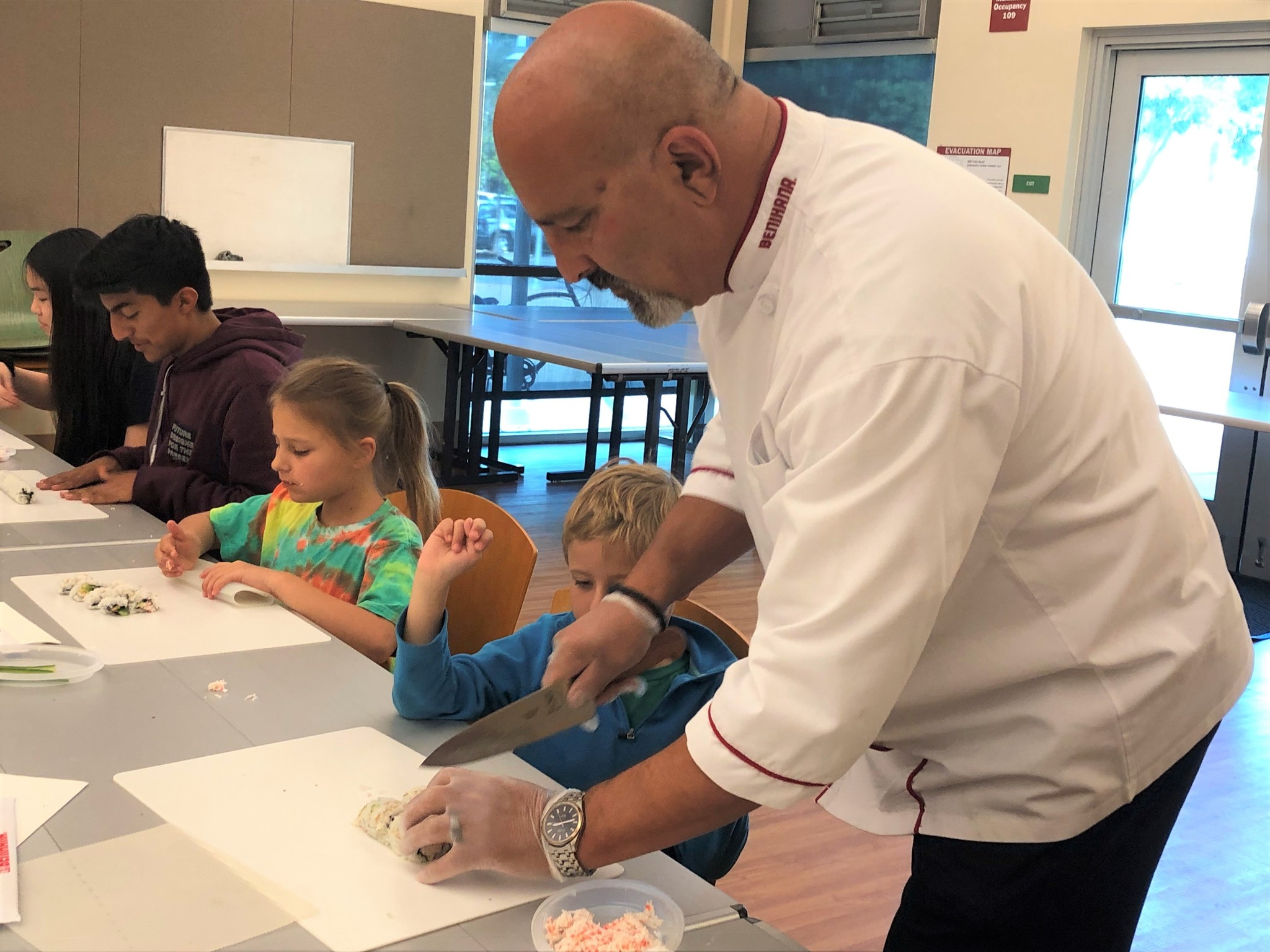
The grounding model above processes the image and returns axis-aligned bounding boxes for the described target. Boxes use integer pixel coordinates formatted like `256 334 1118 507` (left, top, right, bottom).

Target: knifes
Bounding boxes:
420 628 685 769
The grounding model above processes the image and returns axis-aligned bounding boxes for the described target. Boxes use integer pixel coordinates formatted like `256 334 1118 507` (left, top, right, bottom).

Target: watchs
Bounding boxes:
540 792 598 878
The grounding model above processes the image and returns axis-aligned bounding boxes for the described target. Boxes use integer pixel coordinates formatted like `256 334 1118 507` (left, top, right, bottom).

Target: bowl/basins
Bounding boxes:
531 878 685 952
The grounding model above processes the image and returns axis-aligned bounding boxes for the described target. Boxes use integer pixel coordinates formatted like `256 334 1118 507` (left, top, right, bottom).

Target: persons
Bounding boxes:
391 459 751 892
0 227 160 467
392 0 1253 952
153 360 442 664
36 213 306 525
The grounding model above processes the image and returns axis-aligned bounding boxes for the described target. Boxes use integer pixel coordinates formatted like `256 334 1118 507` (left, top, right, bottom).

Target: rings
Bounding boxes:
448 816 464 843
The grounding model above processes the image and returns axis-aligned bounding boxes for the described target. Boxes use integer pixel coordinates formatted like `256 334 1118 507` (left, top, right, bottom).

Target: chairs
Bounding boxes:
0 226 61 372
387 484 536 655
551 586 752 659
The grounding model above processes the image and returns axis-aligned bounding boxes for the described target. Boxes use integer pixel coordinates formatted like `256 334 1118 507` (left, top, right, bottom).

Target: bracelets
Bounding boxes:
0 354 15 377
600 582 668 636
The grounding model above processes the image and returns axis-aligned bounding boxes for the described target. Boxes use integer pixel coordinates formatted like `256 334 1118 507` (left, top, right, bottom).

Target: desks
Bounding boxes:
0 307 806 952
1152 383 1269 436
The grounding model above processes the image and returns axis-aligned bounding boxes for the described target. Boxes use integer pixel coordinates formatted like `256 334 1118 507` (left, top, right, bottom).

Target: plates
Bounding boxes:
0 644 104 688
